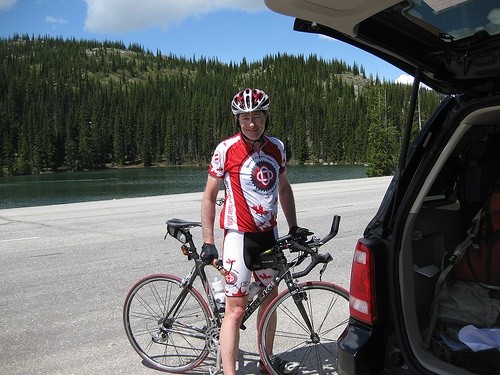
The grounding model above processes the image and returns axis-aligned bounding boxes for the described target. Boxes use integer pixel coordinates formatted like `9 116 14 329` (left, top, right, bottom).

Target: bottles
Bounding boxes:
211 277 225 317
245 281 261 303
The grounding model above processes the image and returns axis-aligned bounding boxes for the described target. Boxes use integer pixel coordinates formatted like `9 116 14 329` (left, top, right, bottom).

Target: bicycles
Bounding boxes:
122 215 350 372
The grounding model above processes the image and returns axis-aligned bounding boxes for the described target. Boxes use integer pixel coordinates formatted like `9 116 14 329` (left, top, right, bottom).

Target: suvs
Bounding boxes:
263 0 499 374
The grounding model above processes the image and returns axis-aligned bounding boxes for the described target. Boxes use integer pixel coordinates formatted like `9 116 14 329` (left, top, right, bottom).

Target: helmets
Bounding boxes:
230 88 271 116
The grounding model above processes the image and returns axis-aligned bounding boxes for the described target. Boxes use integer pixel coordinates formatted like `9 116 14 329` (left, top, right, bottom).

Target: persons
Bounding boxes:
199 87 309 375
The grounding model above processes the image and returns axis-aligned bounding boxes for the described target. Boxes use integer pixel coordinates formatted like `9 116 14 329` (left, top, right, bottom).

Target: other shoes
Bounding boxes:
259 355 300 375
